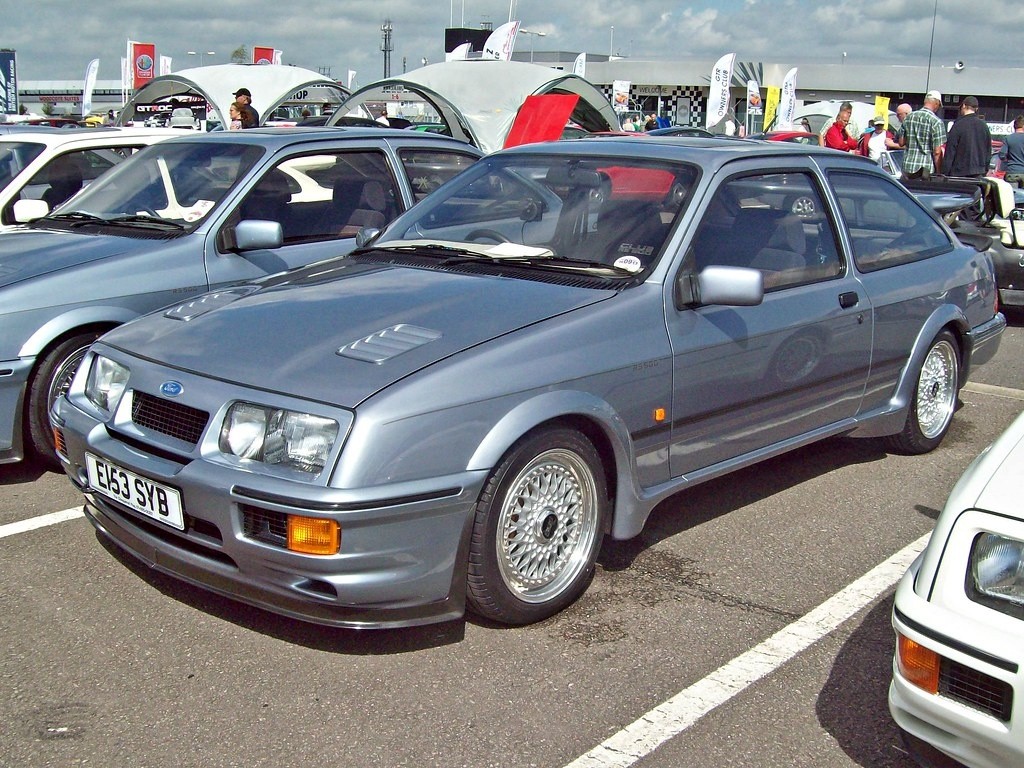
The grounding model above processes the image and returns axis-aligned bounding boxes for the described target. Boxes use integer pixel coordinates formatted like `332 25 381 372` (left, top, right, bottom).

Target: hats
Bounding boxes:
959 96 978 108
873 116 885 125
926 90 942 107
801 120 810 124
232 88 251 97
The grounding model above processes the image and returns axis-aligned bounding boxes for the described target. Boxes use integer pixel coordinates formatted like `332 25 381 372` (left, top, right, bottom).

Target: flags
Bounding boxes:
481 20 521 62
82 59 99 117
705 53 736 129
778 67 798 131
572 52 585 78
446 42 471 60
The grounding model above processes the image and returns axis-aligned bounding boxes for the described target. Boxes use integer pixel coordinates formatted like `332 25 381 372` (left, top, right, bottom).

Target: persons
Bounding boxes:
375 110 390 127
229 102 255 130
232 88 259 128
642 113 660 132
898 89 1024 190
103 110 117 127
321 104 335 115
817 101 913 169
302 108 312 120
796 117 812 143
622 118 642 132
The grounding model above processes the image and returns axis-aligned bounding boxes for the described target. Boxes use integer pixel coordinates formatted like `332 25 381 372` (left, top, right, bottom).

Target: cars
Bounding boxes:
0 123 339 240
549 119 825 212
883 411 1024 768
0 124 568 467
59 126 1007 634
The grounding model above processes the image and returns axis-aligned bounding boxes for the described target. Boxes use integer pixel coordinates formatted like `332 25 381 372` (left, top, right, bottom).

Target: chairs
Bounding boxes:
35 163 83 210
0 161 21 223
573 200 664 267
308 175 386 236
236 170 292 235
712 208 806 271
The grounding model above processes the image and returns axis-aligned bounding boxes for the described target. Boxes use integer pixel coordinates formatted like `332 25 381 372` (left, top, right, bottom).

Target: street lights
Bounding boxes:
610 25 617 62
187 50 215 68
520 28 546 63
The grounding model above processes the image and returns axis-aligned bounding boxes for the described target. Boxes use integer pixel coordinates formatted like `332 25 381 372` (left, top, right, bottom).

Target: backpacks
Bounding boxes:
232 119 257 129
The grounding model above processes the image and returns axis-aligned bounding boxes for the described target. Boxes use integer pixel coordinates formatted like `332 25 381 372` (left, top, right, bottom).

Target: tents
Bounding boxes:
791 100 898 135
324 59 625 191
114 66 375 130
